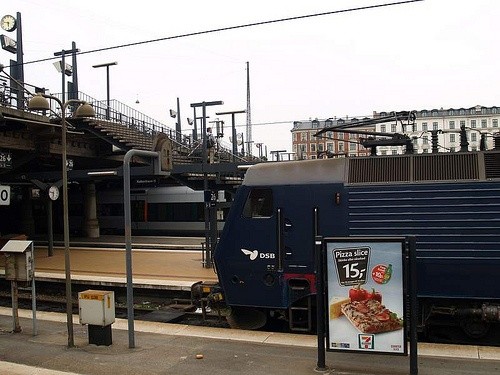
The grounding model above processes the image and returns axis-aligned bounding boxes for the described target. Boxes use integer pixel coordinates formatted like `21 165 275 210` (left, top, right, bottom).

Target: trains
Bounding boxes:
203 113 500 331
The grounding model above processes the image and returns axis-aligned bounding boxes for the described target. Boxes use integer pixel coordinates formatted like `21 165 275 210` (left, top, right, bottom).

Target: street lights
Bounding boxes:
26 92 97 348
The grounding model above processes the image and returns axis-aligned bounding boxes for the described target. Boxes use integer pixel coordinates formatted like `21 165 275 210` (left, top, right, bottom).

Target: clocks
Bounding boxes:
0 15 16 33
48 186 60 201
